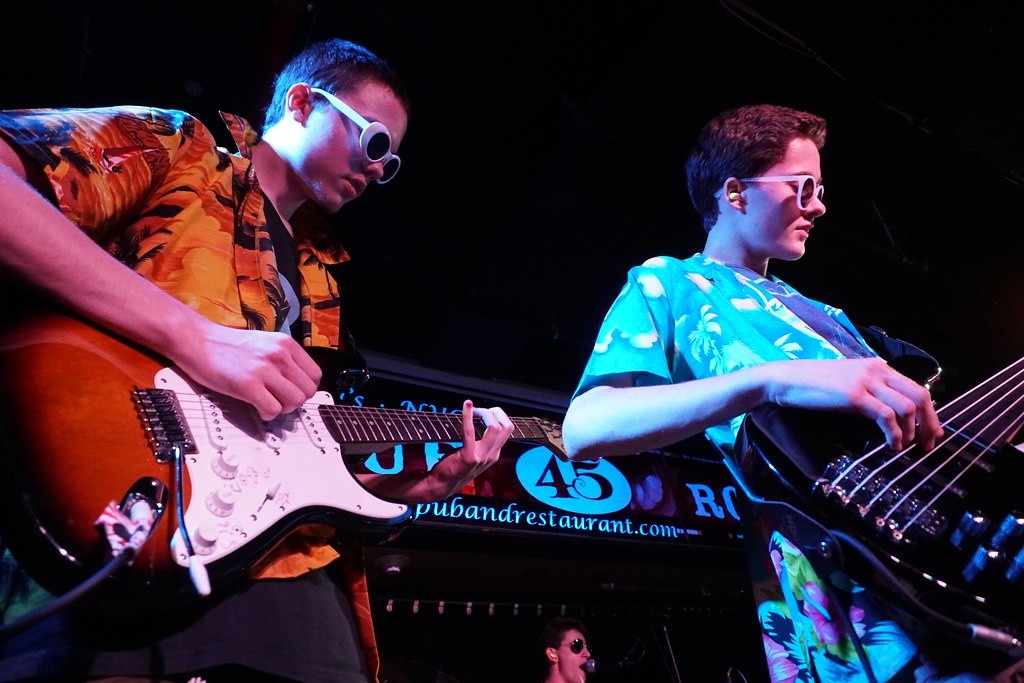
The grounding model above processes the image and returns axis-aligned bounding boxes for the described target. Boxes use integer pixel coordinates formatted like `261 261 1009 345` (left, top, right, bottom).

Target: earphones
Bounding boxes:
730 192 744 203
550 653 556 658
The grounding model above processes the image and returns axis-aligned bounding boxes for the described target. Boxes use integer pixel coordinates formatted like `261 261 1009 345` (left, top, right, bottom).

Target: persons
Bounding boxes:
536 623 593 683
0 41 407 682
562 107 979 683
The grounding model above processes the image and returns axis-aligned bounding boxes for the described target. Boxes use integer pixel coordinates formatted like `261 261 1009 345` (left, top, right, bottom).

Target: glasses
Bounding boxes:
312 88 403 185
553 639 592 654
713 175 826 210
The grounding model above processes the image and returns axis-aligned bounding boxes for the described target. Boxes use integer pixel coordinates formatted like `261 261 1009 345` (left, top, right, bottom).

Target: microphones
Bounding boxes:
585 659 634 673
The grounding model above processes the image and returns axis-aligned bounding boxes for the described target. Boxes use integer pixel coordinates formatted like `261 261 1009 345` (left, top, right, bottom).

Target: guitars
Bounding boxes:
731 357 1023 673
0 301 599 620
